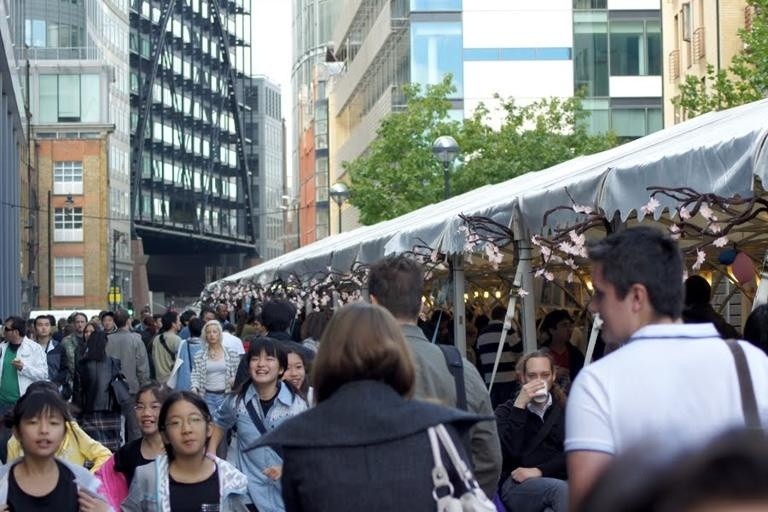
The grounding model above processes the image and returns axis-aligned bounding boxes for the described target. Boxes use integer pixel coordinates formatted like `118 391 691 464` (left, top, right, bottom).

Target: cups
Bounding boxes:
533 381 548 404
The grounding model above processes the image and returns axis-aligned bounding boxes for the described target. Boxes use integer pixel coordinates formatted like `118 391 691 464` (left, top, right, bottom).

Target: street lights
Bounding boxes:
112 231 128 312
328 182 349 233
431 135 461 199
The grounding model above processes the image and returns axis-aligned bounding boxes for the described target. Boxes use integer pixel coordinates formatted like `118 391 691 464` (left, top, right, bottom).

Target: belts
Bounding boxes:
206 389 226 394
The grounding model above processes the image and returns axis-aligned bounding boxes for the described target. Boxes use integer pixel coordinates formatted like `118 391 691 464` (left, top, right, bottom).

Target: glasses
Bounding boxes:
133 404 160 412
165 415 205 428
4 326 13 332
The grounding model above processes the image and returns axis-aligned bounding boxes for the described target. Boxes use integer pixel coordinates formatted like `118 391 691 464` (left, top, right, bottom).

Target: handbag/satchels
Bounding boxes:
111 357 131 406
426 422 498 512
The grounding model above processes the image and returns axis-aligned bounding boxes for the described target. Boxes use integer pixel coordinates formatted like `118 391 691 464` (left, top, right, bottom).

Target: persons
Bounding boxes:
46 303 234 341
6 381 113 475
167 318 204 392
682 275 741 338
152 312 182 384
235 310 253 337
0 388 116 512
114 382 174 489
0 315 48 428
476 305 522 409
74 322 100 370
417 302 489 348
233 299 315 399
206 337 308 512
104 309 152 398
241 303 478 511
540 309 584 382
368 254 502 502
563 226 768 512
190 320 240 419
494 350 571 512
33 315 67 392
303 312 321 352
118 390 252 512
253 314 268 337
59 312 88 377
577 426 768 512
73 330 119 453
